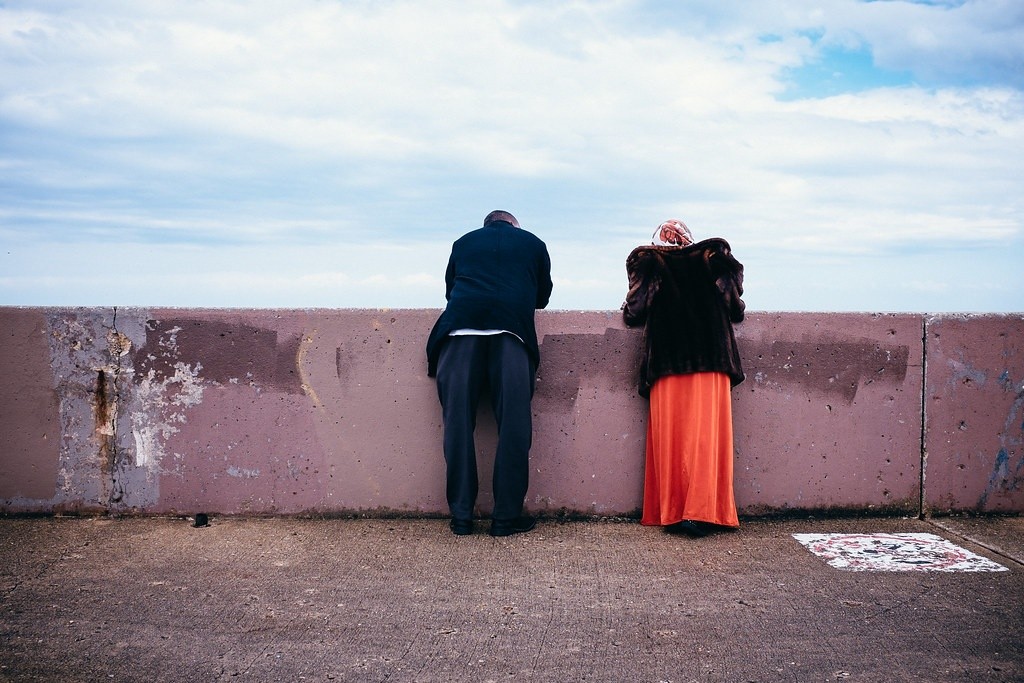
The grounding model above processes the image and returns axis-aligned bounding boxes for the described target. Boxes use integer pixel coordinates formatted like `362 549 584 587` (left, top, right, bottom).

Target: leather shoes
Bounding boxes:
490 514 537 536
450 517 473 535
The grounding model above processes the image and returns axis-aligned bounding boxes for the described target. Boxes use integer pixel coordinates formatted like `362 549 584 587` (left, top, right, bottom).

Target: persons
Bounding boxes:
426 209 553 535
621 219 745 530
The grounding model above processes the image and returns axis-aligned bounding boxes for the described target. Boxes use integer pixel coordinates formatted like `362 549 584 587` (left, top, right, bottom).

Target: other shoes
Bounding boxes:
664 520 709 537
